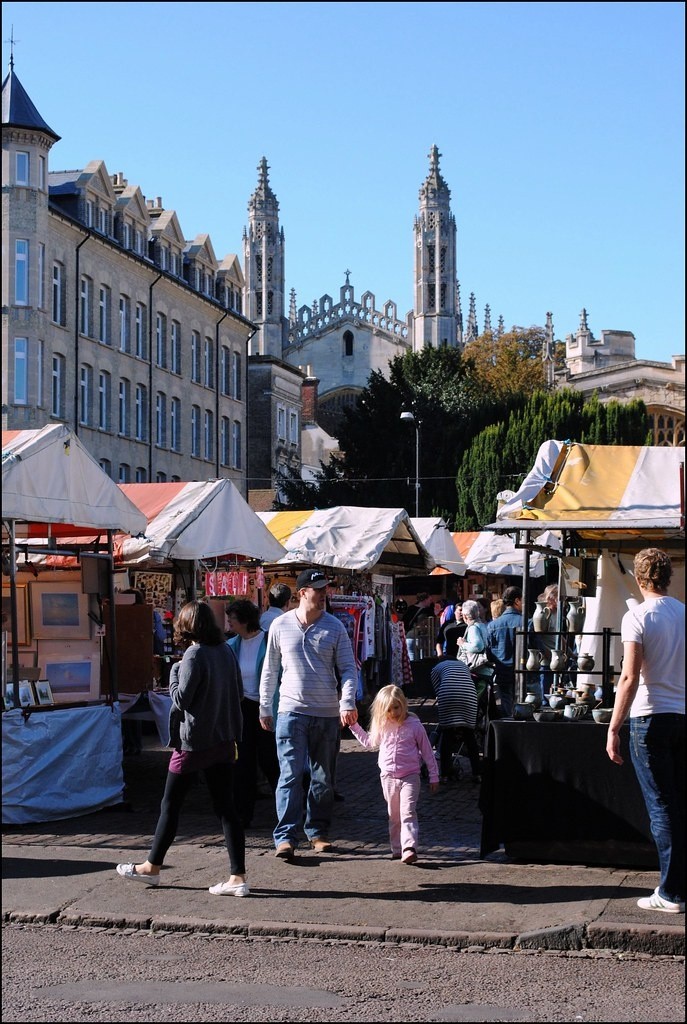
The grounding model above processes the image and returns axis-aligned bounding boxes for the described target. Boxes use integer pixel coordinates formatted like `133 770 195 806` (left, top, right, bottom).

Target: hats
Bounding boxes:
502 586 522 605
296 569 332 588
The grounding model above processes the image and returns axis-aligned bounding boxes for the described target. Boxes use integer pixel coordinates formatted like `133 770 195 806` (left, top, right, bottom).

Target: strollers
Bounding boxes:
423 660 499 785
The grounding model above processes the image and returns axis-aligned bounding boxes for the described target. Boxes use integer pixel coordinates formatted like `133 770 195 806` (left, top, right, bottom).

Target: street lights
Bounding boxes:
400 411 420 518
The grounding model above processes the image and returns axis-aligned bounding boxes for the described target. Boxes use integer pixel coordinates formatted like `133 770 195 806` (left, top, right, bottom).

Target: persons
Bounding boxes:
604 548 686 913
115 601 250 898
124 583 345 830
402 582 588 785
349 683 439 863
260 569 358 859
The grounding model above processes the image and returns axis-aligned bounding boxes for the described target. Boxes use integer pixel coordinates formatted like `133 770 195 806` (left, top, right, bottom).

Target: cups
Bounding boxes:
563 705 578 722
570 703 581 720
579 703 588 718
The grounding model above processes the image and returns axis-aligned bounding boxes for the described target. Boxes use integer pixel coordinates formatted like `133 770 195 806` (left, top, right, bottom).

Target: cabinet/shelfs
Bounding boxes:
515 626 624 710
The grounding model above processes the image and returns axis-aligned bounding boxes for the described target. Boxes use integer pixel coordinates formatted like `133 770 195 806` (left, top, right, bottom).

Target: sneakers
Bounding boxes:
637 886 685 913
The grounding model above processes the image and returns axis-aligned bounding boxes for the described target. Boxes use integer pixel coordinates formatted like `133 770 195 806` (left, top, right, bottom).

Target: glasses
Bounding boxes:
228 617 238 620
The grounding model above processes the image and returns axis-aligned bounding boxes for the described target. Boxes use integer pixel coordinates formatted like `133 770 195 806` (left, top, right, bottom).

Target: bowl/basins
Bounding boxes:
591 708 612 723
532 712 556 722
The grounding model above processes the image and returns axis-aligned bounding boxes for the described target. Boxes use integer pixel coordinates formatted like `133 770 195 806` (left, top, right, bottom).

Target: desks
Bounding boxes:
1 702 122 824
477 717 660 870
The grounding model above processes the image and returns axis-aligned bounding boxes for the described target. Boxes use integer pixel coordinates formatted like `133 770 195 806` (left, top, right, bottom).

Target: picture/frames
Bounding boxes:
1 582 30 646
29 580 91 639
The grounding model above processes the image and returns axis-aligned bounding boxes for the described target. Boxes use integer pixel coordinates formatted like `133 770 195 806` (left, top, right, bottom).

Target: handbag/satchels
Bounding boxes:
465 732 481 761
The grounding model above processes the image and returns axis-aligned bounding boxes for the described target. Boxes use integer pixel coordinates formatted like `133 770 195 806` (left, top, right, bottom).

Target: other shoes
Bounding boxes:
334 792 346 802
258 777 273 799
136 750 141 756
401 851 417 864
393 853 401 858
310 838 332 852
275 842 295 857
439 776 448 784
116 863 160 886
239 819 250 828
435 751 441 759
124 749 135 756
209 882 251 897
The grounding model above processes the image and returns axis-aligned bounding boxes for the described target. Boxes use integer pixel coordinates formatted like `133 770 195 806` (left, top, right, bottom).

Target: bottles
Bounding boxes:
550 649 567 671
526 649 542 671
578 653 595 671
513 702 535 720
533 601 551 632
525 684 596 709
566 602 585 632
